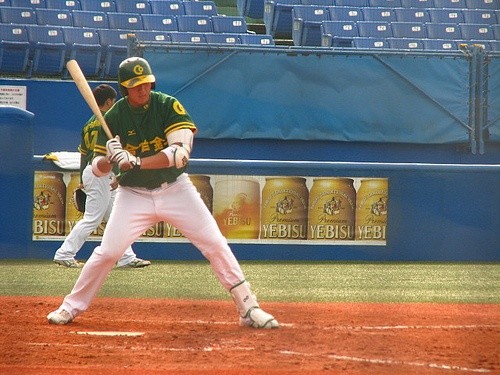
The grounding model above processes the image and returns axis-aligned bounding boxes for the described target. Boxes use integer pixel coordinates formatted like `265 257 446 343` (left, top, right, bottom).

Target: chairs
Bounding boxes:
0 0 500 80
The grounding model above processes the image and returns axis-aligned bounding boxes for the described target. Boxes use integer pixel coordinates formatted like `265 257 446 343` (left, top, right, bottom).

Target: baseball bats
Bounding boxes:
66 58 131 173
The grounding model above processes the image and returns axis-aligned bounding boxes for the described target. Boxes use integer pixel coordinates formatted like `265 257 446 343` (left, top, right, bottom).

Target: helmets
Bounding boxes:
118 57 156 97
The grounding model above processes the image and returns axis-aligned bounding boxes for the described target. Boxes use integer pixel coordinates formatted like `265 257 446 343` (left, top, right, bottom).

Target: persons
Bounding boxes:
47 56 280 330
53 85 151 268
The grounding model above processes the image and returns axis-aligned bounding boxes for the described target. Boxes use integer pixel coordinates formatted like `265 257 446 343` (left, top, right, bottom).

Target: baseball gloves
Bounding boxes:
71 188 87 214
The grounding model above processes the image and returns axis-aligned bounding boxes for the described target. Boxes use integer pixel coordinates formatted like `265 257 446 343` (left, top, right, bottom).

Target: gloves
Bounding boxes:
106 135 122 161
109 150 140 169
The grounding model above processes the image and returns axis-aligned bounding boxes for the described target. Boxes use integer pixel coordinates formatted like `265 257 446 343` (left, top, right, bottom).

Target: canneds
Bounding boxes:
307 178 356 241
65 173 107 237
355 179 388 240
260 177 309 239
33 172 66 236
140 175 213 238
212 177 261 240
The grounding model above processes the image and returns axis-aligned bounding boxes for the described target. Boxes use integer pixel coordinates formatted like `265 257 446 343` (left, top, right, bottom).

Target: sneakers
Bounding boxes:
55 258 84 267
239 310 279 329
47 309 71 326
127 257 151 268
